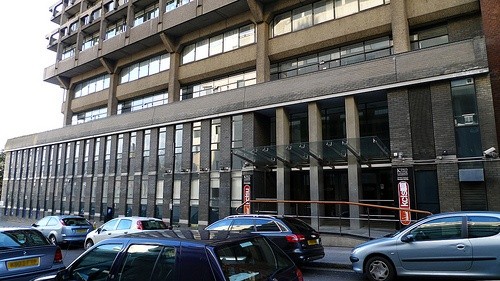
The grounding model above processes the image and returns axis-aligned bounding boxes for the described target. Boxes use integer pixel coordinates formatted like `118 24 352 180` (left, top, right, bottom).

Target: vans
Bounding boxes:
31 230 306 281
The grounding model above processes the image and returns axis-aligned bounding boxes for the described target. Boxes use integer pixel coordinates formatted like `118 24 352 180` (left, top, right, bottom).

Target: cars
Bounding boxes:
84 215 170 251
30 214 94 247
203 214 325 262
350 210 500 280
0 226 66 281
